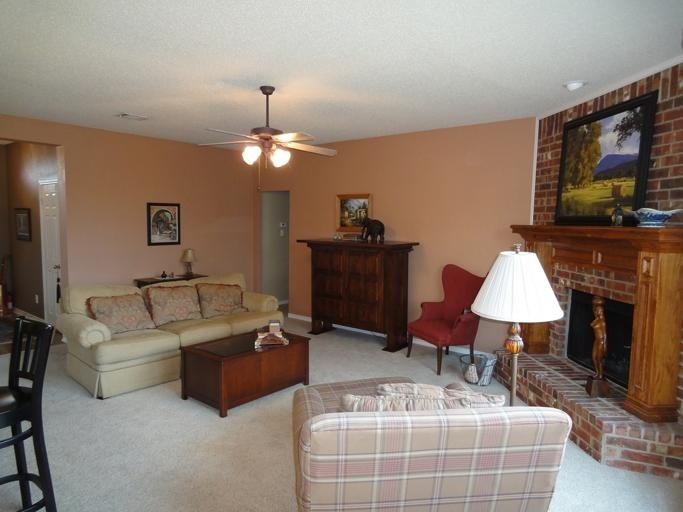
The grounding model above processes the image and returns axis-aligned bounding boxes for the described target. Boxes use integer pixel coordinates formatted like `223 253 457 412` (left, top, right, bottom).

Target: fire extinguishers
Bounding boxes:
5 292 13 315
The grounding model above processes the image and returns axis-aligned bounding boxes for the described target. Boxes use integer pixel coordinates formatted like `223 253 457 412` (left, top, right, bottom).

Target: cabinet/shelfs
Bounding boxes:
289 231 413 353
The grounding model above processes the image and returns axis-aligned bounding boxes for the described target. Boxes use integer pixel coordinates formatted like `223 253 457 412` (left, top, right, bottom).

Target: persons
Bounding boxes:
587 298 611 382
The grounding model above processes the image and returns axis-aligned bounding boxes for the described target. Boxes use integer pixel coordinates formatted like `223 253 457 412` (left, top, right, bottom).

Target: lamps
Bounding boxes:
239 140 291 169
469 245 565 406
177 248 198 277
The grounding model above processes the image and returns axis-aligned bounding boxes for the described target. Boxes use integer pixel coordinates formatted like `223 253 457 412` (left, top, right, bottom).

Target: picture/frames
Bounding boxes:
551 88 662 227
143 199 182 248
12 206 34 243
333 191 374 235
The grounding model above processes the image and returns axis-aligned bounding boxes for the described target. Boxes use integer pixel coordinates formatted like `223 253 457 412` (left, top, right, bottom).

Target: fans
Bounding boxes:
196 83 336 157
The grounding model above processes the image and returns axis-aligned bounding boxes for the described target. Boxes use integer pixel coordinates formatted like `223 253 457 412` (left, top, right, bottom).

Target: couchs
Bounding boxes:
53 270 283 402
279 366 570 512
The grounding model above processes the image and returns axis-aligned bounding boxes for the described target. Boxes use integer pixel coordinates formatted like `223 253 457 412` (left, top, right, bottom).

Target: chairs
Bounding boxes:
404 259 489 381
0 310 61 512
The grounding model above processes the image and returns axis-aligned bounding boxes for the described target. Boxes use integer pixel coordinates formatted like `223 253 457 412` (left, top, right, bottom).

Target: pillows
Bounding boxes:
327 389 508 411
370 380 468 398
83 289 158 339
146 284 201 330
192 278 248 323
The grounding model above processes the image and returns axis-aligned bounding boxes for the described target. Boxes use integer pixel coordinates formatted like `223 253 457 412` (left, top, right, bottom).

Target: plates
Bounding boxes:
621 206 683 228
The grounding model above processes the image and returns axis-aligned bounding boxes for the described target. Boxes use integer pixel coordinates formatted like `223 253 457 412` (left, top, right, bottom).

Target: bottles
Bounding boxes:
611 203 624 226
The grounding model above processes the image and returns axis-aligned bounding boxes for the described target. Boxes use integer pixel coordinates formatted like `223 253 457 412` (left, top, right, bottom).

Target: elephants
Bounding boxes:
361 217 385 245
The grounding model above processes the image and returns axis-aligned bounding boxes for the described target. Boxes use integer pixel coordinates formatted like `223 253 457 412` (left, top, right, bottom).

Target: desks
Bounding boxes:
134 275 205 284
